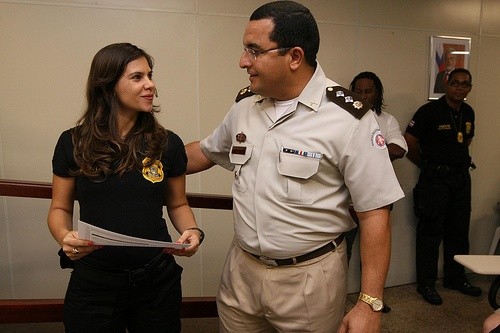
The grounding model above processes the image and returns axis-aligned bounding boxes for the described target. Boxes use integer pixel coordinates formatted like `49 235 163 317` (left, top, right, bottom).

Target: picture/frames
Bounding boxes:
428 35 471 101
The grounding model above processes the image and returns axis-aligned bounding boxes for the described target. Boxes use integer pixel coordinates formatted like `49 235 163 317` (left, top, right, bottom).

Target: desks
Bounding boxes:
454 255 500 274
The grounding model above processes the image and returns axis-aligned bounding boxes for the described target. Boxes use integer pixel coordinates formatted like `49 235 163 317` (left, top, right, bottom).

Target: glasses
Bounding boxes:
448 80 471 88
243 47 292 60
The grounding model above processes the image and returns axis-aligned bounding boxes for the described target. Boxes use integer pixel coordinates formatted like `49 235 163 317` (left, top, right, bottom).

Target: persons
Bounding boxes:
345 71 408 314
434 48 457 94
401 69 483 306
48 43 200 333
182 0 406 333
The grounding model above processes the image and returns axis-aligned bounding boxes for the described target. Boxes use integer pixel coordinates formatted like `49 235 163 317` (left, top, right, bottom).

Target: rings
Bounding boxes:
72 247 80 254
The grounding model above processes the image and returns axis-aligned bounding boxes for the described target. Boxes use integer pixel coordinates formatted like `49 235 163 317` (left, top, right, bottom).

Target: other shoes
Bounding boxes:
416 286 443 305
453 282 482 296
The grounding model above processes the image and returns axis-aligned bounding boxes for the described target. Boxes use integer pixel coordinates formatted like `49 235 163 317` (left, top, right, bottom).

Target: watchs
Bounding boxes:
358 292 384 312
188 228 204 245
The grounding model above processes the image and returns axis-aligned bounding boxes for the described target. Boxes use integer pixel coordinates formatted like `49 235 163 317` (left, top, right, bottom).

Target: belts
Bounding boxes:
243 233 344 266
78 253 170 276
